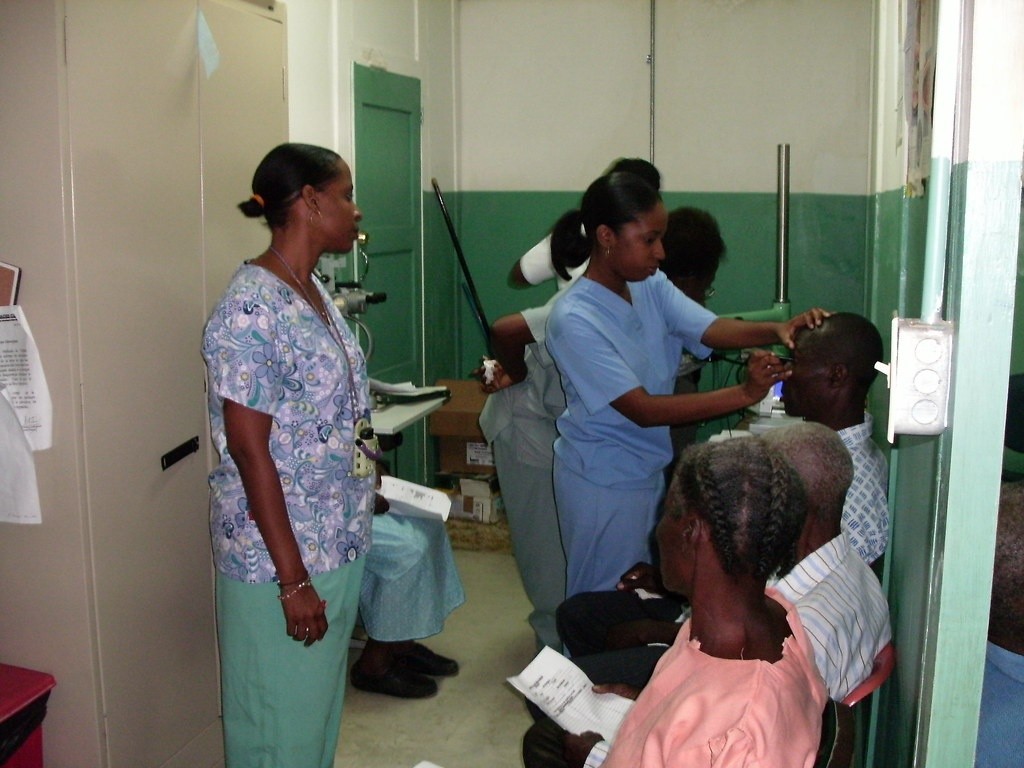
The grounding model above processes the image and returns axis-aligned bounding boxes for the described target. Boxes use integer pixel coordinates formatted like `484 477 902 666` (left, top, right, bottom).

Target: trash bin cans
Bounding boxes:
0 662 57 768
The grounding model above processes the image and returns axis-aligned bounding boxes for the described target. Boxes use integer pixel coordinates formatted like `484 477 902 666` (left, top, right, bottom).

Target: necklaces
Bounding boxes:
319 311 325 315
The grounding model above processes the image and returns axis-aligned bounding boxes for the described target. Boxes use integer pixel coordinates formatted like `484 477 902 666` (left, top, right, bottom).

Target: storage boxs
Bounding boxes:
429 378 505 524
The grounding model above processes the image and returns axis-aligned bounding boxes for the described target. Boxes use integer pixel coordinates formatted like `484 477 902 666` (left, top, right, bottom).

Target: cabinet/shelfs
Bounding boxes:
0 0 288 768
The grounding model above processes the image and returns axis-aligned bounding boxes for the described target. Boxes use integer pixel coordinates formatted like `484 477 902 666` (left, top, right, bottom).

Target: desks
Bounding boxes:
369 388 448 434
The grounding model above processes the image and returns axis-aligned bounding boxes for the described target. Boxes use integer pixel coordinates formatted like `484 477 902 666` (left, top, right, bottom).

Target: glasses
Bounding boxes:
705 286 716 299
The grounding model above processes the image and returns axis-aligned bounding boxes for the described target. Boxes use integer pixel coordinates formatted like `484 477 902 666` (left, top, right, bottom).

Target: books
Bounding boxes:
375 390 451 403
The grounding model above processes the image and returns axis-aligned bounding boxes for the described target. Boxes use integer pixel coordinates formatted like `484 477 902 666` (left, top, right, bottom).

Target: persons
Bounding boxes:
554 313 892 657
351 466 459 699
520 422 892 768
512 158 660 290
600 435 829 768
472 207 727 654
200 143 382 716
546 170 830 658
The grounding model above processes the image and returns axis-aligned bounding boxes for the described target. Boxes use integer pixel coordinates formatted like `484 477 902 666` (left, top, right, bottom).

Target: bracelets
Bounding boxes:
277 571 312 600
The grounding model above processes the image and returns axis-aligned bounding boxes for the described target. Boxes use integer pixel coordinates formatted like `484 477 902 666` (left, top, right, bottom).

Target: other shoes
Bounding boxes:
351 658 437 698
398 641 458 676
